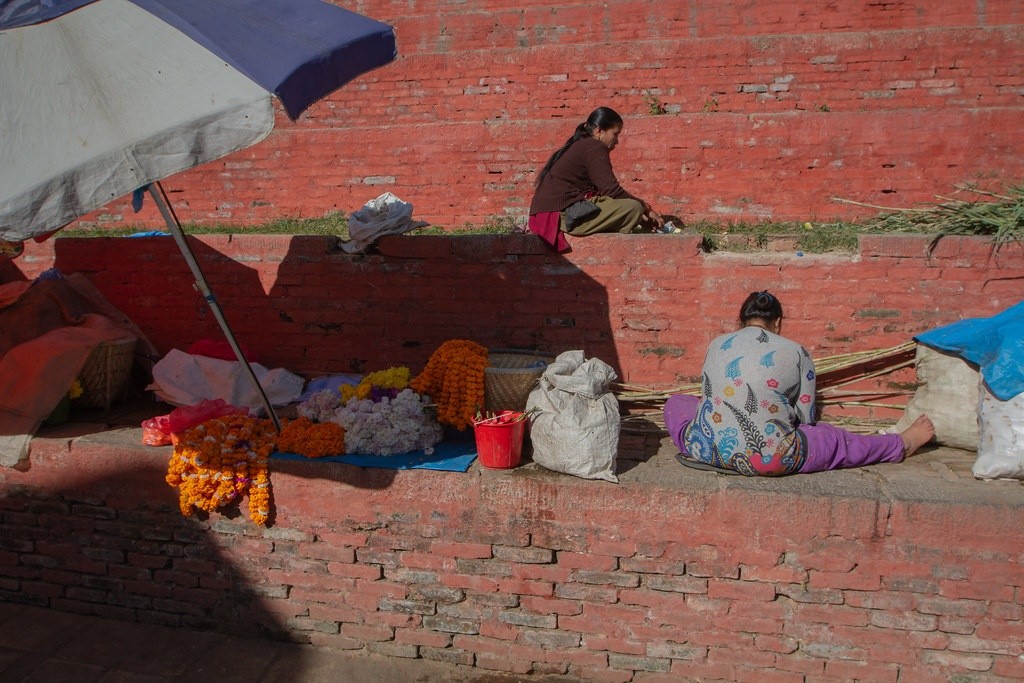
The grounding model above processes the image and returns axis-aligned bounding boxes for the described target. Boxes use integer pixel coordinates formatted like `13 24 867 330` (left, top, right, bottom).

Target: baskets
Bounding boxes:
483 348 565 440
69 337 138 415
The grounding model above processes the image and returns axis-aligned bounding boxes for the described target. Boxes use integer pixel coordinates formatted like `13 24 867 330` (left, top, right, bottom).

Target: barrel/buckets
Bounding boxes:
472 407 537 469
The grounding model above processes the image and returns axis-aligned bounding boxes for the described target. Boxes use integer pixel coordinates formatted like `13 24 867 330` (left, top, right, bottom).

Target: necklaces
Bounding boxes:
743 325 766 329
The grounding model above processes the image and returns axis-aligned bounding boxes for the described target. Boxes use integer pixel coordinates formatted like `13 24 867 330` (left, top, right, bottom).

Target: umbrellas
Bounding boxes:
1 0 398 432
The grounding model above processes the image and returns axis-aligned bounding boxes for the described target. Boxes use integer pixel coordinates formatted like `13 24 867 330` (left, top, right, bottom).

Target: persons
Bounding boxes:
529 106 665 251
665 293 935 477
